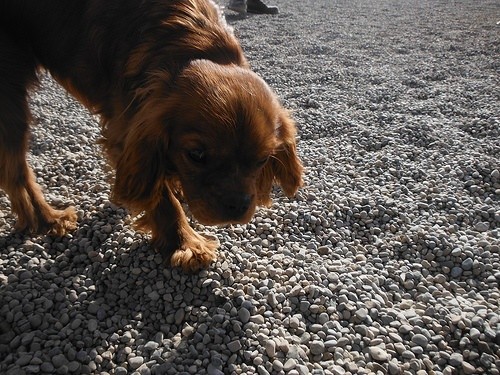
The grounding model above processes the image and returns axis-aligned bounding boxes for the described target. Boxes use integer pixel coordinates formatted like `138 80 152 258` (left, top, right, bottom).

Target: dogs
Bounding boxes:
1 0 304 271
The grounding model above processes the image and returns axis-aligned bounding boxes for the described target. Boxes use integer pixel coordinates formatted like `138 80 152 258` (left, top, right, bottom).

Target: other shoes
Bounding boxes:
246 0 278 14
